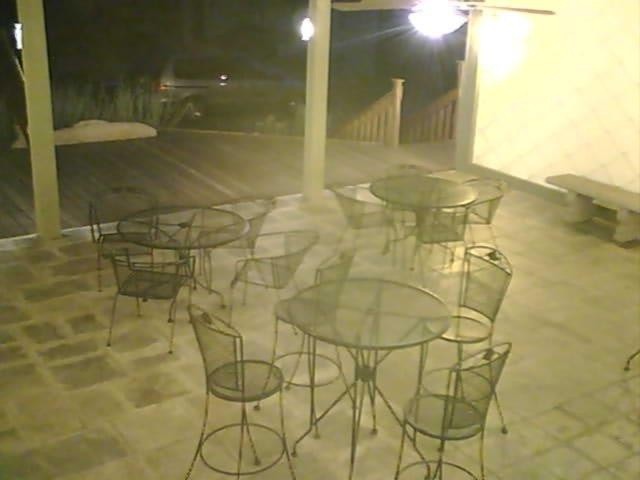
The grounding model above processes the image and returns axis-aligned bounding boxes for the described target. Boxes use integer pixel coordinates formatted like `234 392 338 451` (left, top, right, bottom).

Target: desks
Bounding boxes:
117 204 250 324
287 276 451 479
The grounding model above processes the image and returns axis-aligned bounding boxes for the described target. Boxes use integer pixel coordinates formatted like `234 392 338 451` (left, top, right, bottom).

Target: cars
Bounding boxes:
138 57 248 121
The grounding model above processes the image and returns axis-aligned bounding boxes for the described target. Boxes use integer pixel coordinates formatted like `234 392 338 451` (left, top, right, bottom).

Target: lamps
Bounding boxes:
300 17 314 41
408 0 465 38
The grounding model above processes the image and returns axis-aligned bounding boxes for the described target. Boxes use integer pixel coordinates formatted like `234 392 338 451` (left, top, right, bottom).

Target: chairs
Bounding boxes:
401 245 510 451
229 230 320 336
197 196 277 294
106 251 196 354
185 304 295 480
255 257 358 439
87 186 159 292
328 168 504 271
393 341 510 480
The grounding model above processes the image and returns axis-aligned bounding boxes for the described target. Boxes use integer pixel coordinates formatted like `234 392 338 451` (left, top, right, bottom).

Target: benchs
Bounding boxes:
546 172 640 244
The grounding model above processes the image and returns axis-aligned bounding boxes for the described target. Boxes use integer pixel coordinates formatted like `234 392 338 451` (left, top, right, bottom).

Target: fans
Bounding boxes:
331 0 556 15
